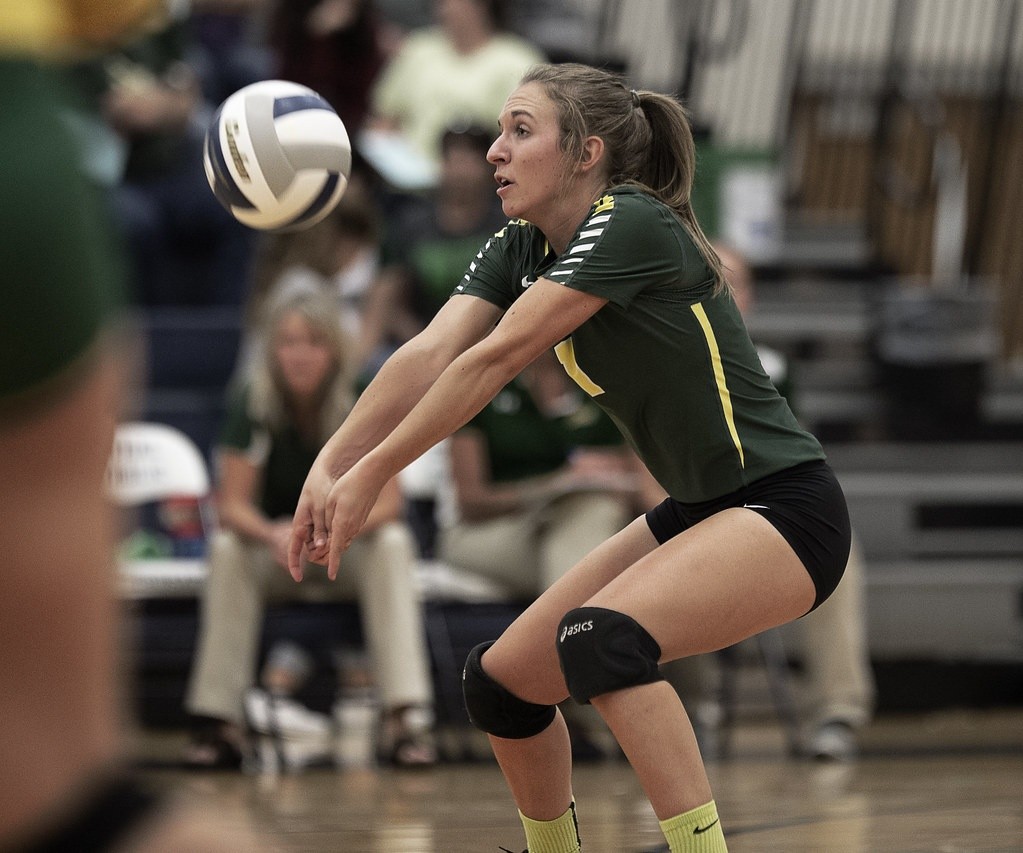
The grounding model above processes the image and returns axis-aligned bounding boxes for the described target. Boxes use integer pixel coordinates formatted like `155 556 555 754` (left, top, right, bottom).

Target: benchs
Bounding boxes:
741 236 1022 657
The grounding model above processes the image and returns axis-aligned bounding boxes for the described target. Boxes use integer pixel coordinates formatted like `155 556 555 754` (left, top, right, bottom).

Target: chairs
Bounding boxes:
100 423 281 776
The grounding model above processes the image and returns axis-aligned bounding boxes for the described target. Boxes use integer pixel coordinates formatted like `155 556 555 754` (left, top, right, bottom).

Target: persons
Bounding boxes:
0 0 878 853
289 64 851 853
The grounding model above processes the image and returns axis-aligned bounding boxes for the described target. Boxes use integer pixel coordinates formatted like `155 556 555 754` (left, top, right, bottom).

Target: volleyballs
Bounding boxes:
203 80 352 234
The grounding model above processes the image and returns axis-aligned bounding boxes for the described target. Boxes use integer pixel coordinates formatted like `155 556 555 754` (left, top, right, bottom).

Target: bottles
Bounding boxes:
333 669 380 781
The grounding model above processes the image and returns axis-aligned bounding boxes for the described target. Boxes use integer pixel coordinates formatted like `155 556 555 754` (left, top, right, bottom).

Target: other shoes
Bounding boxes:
376 725 440 768
810 723 860 762
167 724 244 772
